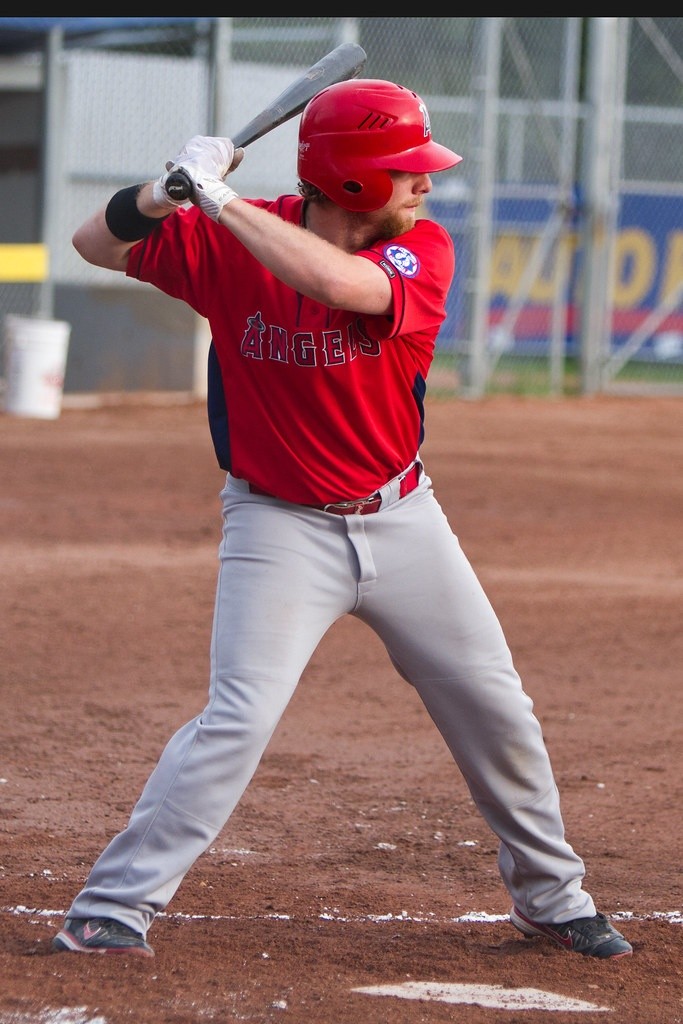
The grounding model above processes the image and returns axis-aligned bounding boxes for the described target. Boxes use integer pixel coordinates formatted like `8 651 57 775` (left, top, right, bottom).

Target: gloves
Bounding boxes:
151 135 245 225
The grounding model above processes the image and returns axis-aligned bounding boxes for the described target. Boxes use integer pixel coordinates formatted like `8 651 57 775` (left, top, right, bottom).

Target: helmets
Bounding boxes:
296 79 462 211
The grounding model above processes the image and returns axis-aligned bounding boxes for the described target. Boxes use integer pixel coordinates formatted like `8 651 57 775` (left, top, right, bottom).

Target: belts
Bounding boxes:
247 463 421 517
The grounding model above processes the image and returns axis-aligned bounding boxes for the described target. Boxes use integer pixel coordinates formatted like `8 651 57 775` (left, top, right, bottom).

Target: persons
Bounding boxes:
48 78 633 961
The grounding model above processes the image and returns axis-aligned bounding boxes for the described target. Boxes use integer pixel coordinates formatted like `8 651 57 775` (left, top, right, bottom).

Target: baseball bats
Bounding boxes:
164 41 368 202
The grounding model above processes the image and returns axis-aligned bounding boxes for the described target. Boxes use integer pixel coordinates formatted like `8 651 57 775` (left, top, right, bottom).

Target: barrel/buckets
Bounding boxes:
6 315 71 421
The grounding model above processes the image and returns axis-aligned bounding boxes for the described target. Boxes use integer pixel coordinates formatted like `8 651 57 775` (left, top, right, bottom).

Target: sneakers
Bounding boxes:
52 911 155 961
510 895 633 960
